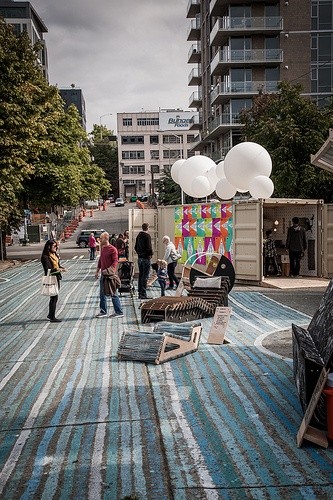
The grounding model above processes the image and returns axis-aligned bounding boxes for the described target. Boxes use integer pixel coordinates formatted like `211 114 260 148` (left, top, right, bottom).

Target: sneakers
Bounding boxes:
109 313 124 318
95 313 107 317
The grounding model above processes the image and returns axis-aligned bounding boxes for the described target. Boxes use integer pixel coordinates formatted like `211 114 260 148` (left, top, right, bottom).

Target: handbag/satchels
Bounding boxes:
170 251 182 261
277 266 281 273
301 251 305 257
103 268 121 296
281 249 290 263
41 269 58 296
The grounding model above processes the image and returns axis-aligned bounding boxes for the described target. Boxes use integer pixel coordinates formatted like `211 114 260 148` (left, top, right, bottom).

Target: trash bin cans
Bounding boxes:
116 260 135 297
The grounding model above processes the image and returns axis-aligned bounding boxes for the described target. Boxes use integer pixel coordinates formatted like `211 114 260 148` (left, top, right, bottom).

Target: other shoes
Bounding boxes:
47 315 50 319
176 285 178 289
265 275 269 277
138 295 140 298
50 318 61 322
165 287 173 290
140 295 153 299
295 275 302 278
276 274 281 277
291 275 293 277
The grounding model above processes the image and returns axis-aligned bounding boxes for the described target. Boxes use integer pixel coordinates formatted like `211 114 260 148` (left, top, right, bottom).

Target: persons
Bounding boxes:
95 232 124 318
157 260 169 296
134 223 153 299
162 236 179 290
286 217 308 278
263 230 281 277
90 230 129 261
41 241 66 323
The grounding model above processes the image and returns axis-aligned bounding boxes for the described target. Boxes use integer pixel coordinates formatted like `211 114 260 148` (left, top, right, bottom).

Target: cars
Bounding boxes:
131 196 138 202
115 198 125 207
141 194 148 202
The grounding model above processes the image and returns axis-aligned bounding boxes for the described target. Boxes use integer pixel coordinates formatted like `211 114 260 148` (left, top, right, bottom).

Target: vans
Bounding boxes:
108 194 115 203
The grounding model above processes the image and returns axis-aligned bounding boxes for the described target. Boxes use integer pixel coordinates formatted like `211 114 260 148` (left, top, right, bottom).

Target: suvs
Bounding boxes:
76 229 113 249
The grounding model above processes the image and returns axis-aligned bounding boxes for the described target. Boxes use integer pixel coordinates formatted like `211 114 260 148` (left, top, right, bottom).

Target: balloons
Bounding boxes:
171 142 274 199
